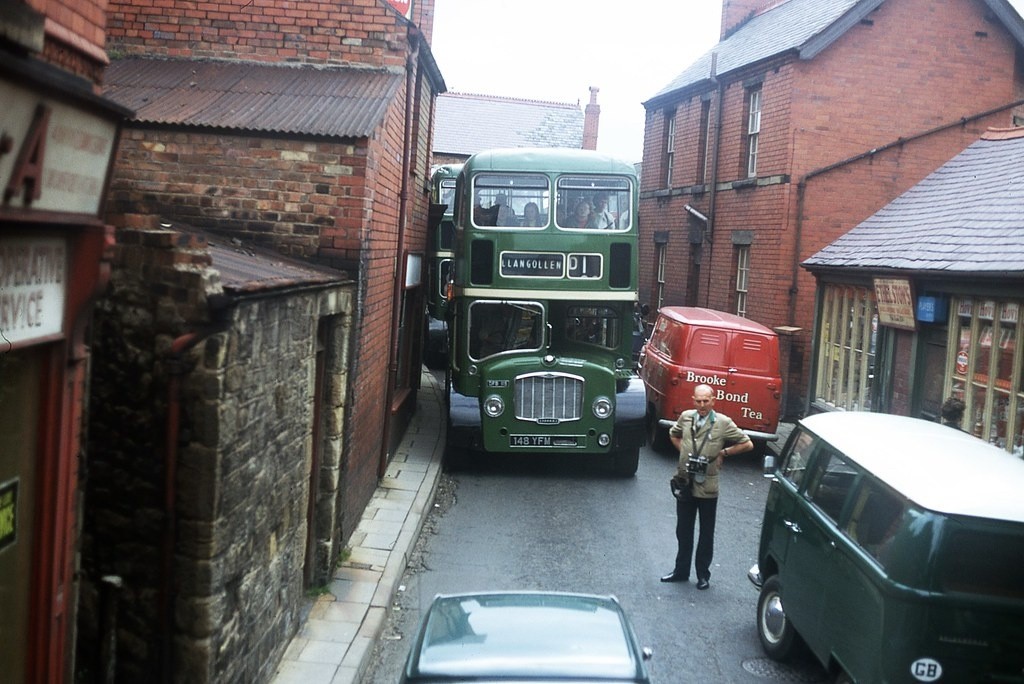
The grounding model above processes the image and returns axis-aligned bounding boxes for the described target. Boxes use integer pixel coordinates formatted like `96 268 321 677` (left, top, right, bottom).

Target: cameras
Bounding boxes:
685 455 709 475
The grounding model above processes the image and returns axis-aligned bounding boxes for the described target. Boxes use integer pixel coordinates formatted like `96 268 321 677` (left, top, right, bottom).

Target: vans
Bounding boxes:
635 305 782 463
746 411 1023 684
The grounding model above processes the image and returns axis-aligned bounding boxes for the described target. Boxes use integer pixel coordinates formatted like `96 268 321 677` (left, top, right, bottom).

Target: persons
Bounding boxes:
489 205 520 227
592 192 615 229
563 200 599 228
660 384 753 590
520 202 544 227
495 193 514 215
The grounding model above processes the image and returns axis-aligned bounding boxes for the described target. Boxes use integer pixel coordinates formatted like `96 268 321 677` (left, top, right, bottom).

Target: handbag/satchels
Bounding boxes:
670 477 691 503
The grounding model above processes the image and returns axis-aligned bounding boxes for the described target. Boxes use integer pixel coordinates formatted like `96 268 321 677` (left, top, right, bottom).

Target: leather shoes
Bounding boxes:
696 577 709 590
660 572 688 582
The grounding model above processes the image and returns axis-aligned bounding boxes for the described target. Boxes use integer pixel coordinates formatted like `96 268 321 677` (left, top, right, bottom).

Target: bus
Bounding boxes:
433 149 652 480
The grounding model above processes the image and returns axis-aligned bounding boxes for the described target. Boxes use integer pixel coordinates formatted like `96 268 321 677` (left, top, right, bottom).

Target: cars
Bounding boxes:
399 592 653 684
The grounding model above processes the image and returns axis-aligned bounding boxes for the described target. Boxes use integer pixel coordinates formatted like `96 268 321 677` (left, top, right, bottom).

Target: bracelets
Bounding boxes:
724 449 727 455
